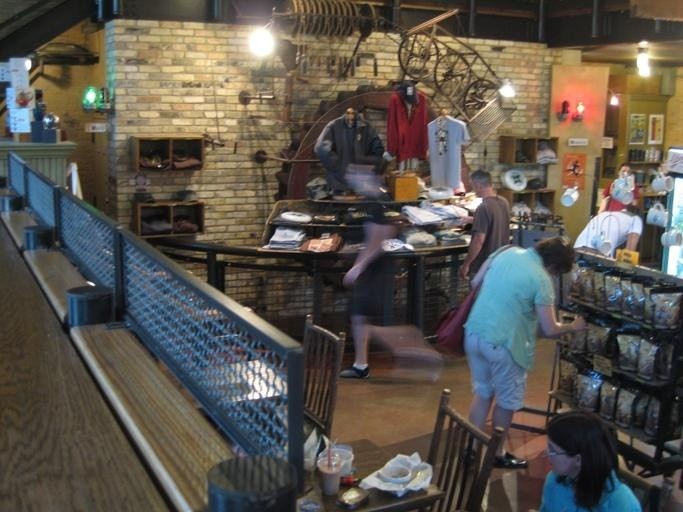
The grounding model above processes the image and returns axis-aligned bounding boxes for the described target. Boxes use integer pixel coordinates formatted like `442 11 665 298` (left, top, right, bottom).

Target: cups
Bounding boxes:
316 457 342 498
318 449 354 478
649 172 673 193
628 147 660 162
608 178 634 205
660 231 682 248
560 185 580 207
635 169 643 184
644 202 668 230
589 232 612 254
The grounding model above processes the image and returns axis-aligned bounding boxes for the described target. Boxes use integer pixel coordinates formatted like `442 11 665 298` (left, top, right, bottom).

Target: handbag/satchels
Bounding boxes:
439 293 482 356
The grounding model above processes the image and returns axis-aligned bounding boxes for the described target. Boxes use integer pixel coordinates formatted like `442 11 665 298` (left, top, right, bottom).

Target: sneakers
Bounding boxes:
341 364 371 378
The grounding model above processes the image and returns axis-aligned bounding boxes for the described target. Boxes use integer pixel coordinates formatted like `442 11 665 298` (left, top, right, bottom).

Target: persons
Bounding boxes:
572 206 643 260
536 409 644 512
596 160 641 215
462 236 588 471
460 168 509 290
338 201 399 379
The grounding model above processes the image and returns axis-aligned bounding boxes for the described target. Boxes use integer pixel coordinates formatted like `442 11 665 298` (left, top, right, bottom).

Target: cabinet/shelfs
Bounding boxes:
545 246 683 494
503 189 555 215
600 134 617 183
130 199 204 241
128 131 204 171
261 195 478 248
629 159 667 267
499 134 559 167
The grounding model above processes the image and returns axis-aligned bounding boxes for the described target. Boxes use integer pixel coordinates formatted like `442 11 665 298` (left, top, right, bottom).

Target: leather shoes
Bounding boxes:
495 451 528 469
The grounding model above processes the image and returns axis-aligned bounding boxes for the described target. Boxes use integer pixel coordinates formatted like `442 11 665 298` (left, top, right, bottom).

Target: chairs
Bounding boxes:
238 314 347 456
613 468 674 512
417 385 503 512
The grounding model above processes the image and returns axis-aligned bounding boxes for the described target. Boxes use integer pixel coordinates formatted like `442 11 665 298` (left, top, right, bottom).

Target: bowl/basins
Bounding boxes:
378 466 412 484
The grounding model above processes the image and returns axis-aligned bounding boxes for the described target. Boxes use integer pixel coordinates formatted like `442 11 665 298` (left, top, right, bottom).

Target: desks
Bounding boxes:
304 441 447 510
0 220 175 512
0 135 77 192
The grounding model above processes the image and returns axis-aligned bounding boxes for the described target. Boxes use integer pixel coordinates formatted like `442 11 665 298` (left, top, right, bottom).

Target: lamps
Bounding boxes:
80 81 109 107
635 40 648 70
606 87 620 106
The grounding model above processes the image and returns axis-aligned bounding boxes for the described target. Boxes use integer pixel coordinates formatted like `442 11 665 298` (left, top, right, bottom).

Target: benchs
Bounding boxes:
21 188 121 324
67 230 304 512
0 148 57 252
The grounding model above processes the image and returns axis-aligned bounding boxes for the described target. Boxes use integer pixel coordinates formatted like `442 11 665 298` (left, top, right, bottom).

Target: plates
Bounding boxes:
501 168 527 192
